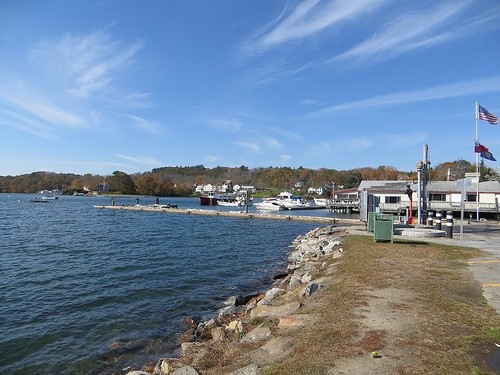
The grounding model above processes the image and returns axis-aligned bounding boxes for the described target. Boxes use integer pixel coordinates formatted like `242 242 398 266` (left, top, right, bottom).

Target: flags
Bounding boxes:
480 151 497 161
474 139 488 153
478 105 499 125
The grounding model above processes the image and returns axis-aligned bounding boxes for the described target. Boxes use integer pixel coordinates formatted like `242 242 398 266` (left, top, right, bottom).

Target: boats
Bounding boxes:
252 192 329 211
41 196 58 200
33 199 49 203
216 199 242 207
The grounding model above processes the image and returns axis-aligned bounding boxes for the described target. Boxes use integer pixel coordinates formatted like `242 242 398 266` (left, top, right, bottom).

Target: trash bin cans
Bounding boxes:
373 214 394 243
368 213 382 234
